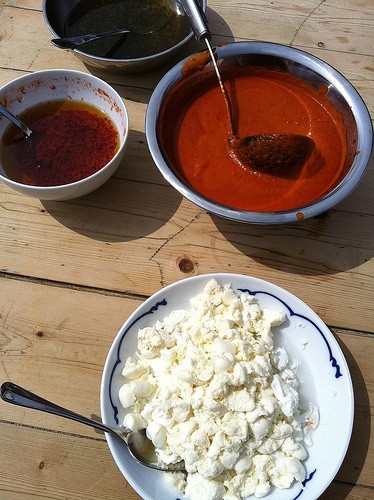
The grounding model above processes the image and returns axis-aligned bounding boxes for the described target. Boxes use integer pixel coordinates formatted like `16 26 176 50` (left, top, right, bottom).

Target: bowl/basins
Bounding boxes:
145 40 373 225
41 0 207 72
0 69 129 201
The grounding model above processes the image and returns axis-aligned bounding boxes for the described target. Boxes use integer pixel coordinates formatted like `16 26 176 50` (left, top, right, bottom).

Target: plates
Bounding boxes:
100 273 353 500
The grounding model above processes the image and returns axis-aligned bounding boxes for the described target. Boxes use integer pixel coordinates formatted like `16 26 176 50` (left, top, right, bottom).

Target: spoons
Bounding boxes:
50 9 174 50
0 105 62 164
0 381 186 471
